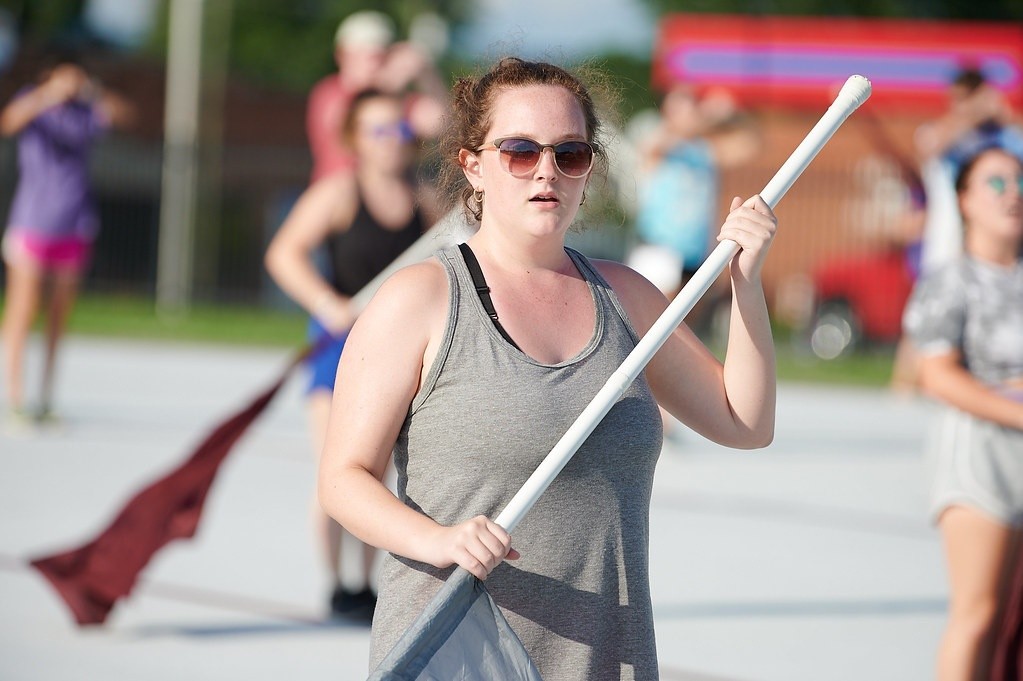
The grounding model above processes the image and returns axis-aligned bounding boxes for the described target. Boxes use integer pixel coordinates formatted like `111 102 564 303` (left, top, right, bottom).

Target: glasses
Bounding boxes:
979 177 1023 195
474 136 599 178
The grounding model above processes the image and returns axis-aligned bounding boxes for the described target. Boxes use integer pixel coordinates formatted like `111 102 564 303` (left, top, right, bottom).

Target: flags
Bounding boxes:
34 388 271 625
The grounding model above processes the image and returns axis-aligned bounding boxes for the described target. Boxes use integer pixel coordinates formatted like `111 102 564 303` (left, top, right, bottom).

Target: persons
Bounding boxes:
0 65 128 412
639 82 764 338
319 54 777 681
265 85 435 626
901 142 1023 681
906 66 1023 195
307 13 445 184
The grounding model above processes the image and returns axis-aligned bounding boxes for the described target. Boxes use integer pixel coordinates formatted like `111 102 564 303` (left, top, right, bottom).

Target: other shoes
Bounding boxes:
331 586 379 624
